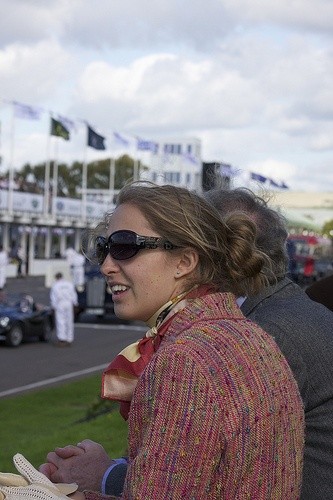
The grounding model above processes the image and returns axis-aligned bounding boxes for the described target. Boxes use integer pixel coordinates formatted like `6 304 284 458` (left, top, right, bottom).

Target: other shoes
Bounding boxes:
52 340 73 349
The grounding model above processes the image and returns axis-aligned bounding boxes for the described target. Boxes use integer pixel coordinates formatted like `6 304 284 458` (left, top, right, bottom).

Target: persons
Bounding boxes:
0 180 306 500
14 293 38 314
40 189 333 500
50 271 78 346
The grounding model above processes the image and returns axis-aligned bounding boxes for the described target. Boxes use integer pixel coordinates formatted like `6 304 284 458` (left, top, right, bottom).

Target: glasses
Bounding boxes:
92 229 186 266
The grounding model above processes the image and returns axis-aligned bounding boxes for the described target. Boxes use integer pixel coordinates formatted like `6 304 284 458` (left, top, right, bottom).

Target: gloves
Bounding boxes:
0 452 79 500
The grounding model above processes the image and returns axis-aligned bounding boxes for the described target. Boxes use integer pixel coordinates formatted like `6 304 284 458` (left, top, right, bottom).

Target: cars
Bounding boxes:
0 292 55 349
